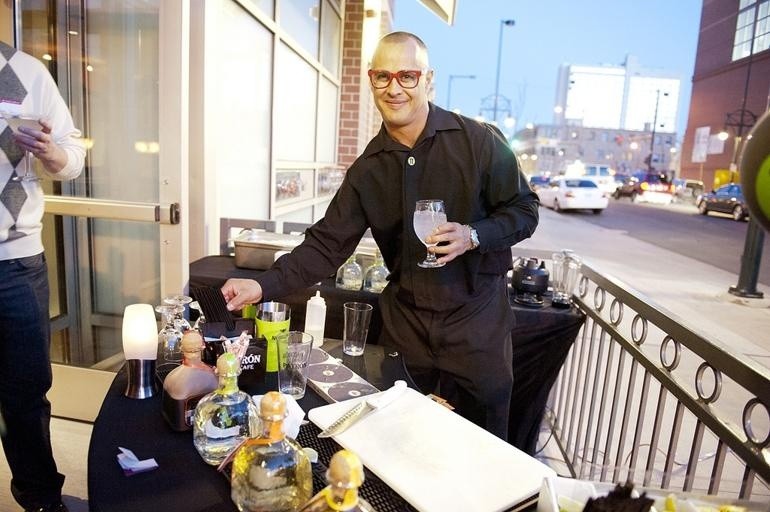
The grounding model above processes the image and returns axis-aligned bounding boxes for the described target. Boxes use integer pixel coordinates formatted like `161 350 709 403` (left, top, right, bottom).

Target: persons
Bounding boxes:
218 30 541 444
0 38 88 512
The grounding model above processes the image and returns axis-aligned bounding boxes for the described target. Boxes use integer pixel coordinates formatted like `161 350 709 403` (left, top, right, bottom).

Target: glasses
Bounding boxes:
369 69 422 89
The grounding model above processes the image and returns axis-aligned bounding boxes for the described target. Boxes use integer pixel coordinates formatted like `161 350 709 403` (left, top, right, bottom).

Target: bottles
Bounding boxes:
335 248 363 291
363 246 393 292
297 449 375 511
229 391 312 510
302 290 327 347
160 331 218 428
193 354 259 466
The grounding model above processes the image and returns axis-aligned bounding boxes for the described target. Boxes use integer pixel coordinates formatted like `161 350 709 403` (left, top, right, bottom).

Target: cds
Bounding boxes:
301 364 353 382
289 345 329 364
328 382 378 402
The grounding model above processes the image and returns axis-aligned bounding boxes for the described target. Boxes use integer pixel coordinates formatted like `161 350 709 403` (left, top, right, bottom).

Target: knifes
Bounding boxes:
318 379 408 440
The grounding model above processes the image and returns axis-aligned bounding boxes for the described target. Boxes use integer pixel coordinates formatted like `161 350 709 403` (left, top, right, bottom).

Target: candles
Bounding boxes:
123 303 157 360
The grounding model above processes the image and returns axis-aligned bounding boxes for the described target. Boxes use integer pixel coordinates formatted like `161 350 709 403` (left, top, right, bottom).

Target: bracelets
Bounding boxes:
462 223 481 251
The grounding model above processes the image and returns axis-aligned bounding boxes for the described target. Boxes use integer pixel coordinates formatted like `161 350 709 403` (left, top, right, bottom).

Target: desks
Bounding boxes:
86 320 541 511
189 256 586 457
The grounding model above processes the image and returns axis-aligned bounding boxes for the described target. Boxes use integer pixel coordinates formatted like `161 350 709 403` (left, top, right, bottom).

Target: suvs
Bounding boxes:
611 172 676 205
673 178 704 200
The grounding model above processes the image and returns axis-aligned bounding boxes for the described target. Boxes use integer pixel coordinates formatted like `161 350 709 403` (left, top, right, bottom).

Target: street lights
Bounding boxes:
476 19 515 127
718 109 757 181
447 74 477 110
647 89 668 170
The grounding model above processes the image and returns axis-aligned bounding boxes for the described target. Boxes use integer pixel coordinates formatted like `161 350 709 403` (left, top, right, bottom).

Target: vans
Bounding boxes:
567 163 613 197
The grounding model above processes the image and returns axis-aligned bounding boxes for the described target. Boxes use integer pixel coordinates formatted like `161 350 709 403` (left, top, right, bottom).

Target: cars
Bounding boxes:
531 176 550 190
535 175 609 215
696 182 750 222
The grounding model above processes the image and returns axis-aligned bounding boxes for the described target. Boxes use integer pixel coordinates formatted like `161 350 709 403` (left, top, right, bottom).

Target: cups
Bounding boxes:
250 392 306 441
276 330 314 400
254 302 291 372
343 302 372 357
552 254 582 309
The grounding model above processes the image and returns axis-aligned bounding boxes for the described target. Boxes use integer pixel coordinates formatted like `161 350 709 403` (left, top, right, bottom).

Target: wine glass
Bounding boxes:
411 198 447 270
3 113 52 181
157 292 207 362
